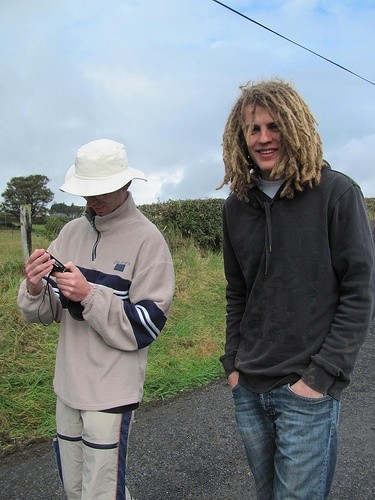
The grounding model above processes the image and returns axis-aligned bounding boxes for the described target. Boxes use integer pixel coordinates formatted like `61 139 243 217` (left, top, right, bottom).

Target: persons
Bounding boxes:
16 139 175 500
217 78 375 500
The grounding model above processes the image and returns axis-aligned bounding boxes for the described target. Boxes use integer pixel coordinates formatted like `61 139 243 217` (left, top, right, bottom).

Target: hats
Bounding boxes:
59 139 148 196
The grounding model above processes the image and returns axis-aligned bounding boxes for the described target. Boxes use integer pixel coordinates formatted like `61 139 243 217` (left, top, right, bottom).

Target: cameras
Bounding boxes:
46 252 67 273
